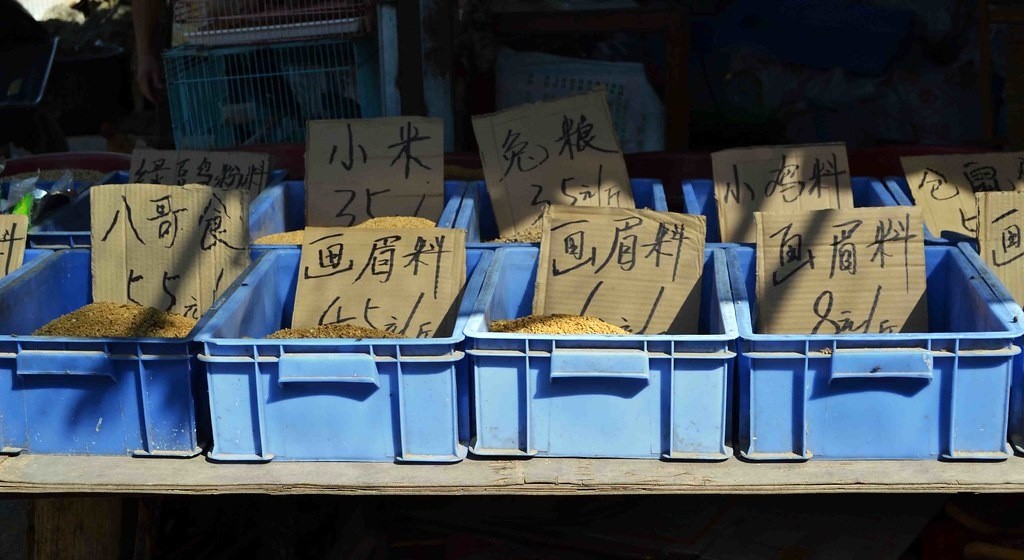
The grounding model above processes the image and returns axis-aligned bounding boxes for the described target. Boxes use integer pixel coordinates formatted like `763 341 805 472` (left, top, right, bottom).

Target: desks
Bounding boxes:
0 457 1024 560
489 0 689 152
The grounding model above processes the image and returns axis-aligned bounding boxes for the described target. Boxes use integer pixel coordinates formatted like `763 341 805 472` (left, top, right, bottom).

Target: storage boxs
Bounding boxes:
493 46 665 152
0 143 1024 460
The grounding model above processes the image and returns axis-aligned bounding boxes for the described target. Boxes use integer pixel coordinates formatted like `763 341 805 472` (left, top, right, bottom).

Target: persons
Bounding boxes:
133 0 265 101
